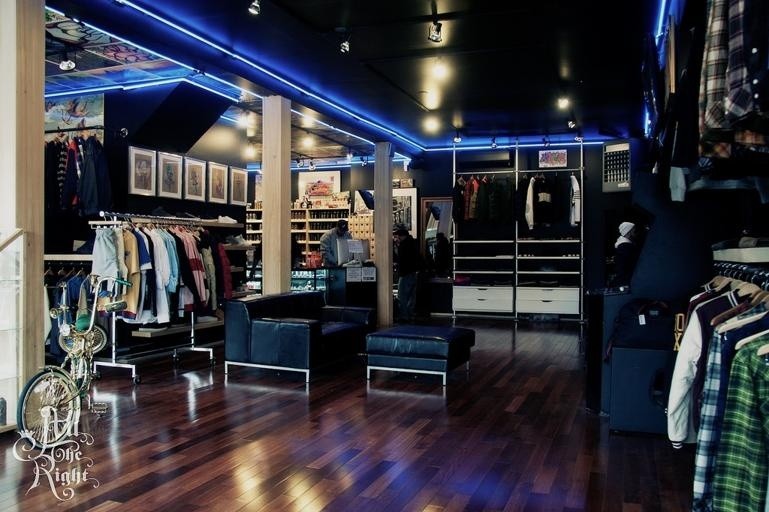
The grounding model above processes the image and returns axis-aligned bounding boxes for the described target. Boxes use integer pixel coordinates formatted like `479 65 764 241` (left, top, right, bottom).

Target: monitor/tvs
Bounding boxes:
338 239 370 268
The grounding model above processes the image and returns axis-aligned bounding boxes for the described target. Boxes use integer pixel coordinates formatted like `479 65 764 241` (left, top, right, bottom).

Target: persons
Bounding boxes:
249 241 262 279
393 228 421 324
434 232 453 277
320 220 353 268
605 221 637 288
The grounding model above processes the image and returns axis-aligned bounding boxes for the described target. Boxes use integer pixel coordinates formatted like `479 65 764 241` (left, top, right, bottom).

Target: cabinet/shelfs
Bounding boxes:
451 142 586 332
196 217 259 300
246 206 351 269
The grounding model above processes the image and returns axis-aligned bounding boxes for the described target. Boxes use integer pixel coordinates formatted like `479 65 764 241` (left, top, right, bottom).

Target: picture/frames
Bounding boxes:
127 145 251 208
353 188 418 239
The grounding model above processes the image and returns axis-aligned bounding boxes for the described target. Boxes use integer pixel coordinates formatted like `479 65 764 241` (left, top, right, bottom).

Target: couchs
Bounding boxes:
223 288 376 394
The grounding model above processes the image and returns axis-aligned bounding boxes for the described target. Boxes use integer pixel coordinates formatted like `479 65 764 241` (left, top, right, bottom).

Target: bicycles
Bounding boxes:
17 276 133 450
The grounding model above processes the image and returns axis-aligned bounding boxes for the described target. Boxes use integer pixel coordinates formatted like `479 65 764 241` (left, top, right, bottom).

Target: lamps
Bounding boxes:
568 120 577 129
307 162 318 171
344 146 354 165
247 0 263 16
57 46 77 72
338 32 354 54
427 20 444 45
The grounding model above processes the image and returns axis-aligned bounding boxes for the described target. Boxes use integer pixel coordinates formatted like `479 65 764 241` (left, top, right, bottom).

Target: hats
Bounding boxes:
618 221 635 237
337 220 348 231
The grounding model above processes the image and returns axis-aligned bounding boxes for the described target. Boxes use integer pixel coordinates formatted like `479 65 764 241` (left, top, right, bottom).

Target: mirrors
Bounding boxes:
420 195 453 285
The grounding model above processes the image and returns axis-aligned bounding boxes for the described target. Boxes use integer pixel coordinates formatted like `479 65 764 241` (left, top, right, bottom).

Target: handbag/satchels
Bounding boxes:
610 297 674 349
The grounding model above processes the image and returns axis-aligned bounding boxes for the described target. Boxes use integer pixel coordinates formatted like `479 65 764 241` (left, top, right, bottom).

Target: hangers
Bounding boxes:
456 167 576 181
690 245 769 359
44 123 101 150
41 260 94 289
88 211 208 239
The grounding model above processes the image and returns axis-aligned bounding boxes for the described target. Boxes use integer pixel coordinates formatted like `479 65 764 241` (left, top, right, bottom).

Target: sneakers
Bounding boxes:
218 216 237 224
